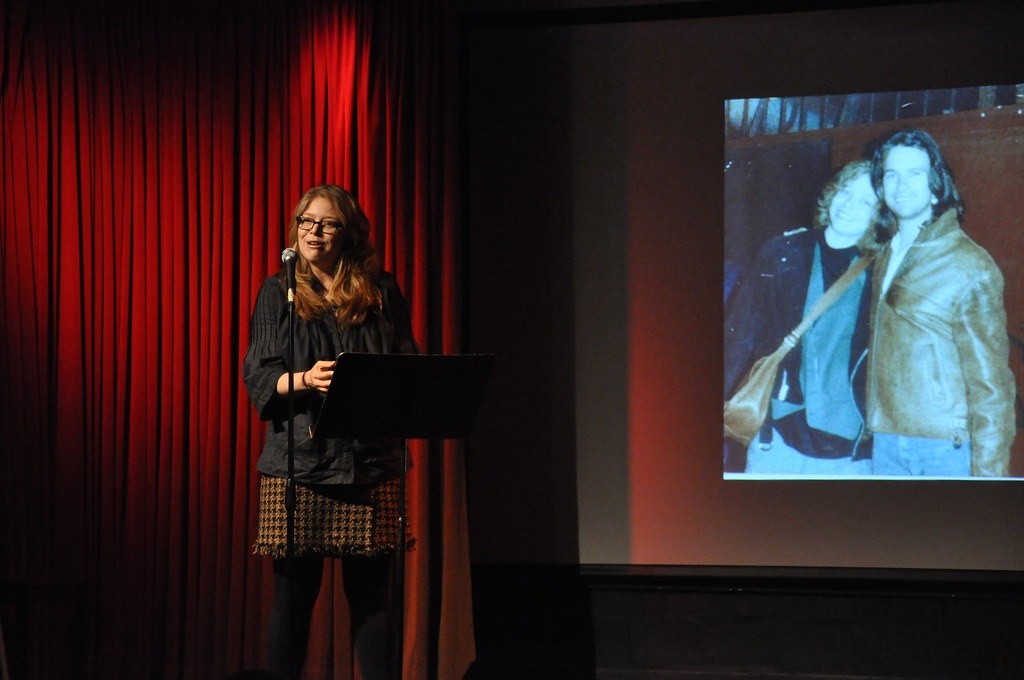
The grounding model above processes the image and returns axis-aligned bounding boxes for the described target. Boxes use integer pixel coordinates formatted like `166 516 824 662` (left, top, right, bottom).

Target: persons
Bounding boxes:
722 130 1016 478
242 184 421 680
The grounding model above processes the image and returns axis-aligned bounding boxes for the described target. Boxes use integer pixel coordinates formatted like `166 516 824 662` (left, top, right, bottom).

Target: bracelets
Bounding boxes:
302 372 309 390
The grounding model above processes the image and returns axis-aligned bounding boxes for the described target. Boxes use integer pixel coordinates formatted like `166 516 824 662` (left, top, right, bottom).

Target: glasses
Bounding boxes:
295 215 344 235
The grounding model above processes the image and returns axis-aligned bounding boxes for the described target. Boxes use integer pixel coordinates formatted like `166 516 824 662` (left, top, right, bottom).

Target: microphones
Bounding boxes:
282 248 300 306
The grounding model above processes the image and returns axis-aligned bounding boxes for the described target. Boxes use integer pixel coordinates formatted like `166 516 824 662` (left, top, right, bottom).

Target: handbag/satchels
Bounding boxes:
723 356 777 446
